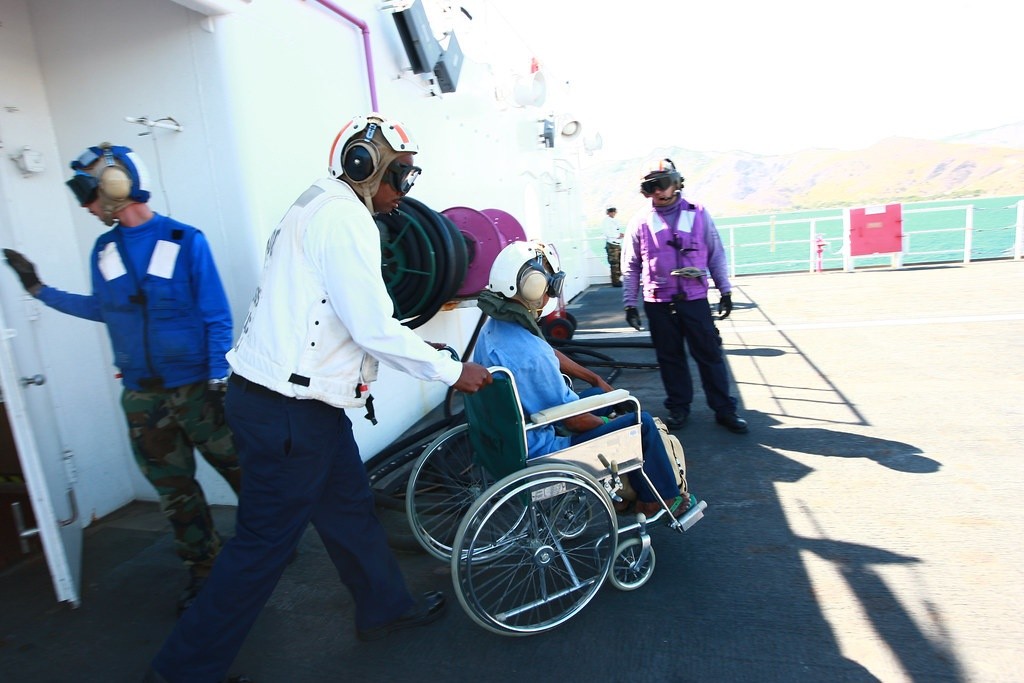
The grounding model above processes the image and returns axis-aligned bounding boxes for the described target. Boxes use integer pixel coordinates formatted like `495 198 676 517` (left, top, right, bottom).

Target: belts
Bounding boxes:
232 374 323 404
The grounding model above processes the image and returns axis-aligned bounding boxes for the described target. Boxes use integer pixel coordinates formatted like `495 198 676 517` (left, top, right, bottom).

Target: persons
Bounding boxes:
623 155 749 434
140 113 492 683
605 205 626 288
469 240 696 530
3 143 242 609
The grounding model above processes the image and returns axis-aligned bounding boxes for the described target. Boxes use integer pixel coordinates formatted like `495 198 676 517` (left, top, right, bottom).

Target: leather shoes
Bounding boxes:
358 588 449 641
666 411 688 429
716 411 749 432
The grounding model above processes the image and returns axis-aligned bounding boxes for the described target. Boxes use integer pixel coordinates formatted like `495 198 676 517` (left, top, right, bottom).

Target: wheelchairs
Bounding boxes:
405 339 708 638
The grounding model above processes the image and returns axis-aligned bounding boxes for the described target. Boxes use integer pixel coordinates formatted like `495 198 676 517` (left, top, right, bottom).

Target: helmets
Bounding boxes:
328 111 417 179
73 146 149 202
641 158 682 191
485 239 560 298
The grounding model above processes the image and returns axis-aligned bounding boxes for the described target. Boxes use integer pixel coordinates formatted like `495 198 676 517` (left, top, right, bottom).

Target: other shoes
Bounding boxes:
613 280 623 287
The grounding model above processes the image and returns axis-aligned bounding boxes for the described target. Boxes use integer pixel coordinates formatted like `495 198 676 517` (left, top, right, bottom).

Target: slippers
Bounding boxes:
647 494 697 525
617 499 637 521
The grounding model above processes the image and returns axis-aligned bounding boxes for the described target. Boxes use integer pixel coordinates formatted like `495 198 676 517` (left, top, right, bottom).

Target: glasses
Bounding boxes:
86 188 98 203
553 271 567 297
642 177 671 194
394 166 422 194
67 176 95 205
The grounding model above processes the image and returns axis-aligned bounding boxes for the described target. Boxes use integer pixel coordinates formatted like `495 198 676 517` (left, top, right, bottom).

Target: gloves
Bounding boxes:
4 249 39 290
719 294 732 320
627 307 646 332
198 383 227 427
